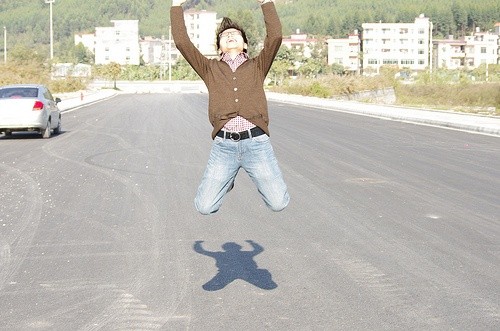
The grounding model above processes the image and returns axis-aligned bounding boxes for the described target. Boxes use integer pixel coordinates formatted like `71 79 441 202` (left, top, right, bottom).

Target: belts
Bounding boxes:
216 126 265 142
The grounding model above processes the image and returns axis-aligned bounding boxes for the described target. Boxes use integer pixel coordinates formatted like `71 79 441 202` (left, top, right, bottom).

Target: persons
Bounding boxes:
170 0 291 215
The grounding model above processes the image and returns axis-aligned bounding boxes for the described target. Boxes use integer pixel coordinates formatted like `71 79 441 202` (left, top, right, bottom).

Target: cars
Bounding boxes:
0 84 62 138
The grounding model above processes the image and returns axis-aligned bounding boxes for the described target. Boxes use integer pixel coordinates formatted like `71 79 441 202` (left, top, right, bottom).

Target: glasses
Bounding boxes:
219 31 241 38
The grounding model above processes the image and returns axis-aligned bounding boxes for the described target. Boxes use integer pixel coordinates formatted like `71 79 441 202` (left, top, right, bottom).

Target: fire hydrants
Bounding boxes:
80 92 84 100
